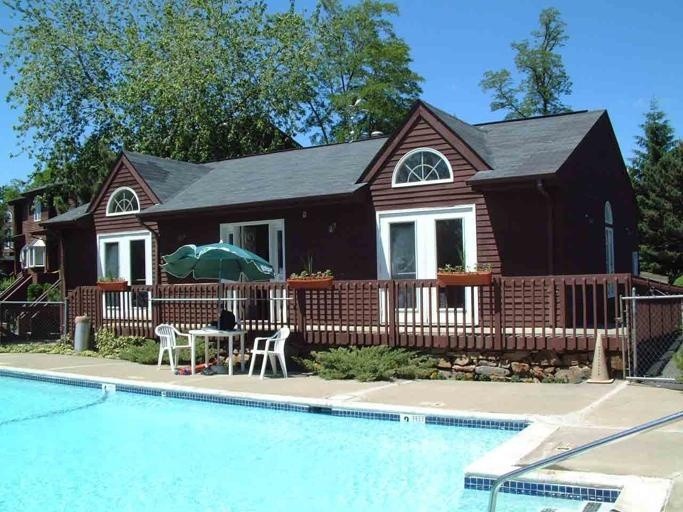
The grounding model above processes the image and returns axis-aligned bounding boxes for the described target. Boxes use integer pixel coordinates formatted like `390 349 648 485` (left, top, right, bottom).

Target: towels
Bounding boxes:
74 316 91 352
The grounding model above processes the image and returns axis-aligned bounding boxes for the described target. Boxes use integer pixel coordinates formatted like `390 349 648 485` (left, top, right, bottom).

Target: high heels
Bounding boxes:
584 333 614 385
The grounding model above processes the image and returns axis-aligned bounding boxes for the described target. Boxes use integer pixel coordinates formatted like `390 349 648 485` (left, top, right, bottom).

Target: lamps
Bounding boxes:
153 323 291 379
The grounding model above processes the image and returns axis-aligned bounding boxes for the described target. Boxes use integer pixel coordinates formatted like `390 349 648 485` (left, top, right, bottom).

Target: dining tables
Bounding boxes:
290 270 333 290
438 264 495 288
95 271 127 292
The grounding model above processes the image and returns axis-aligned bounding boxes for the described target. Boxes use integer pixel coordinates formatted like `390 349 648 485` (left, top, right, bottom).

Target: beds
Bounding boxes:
156 239 274 330
